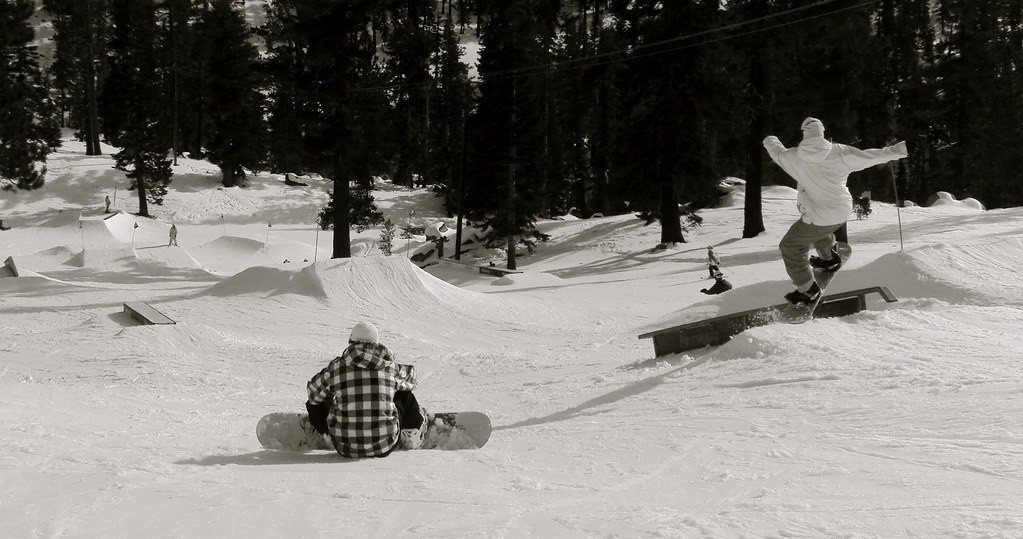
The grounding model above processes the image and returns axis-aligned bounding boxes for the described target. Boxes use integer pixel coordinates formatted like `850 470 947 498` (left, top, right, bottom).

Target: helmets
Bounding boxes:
713 271 723 277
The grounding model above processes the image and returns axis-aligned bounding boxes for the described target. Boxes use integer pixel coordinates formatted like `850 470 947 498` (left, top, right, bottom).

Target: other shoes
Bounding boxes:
810 250 840 270
785 282 821 304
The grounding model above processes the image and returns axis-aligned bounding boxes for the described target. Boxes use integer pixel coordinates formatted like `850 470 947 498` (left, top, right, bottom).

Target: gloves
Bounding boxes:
700 288 706 293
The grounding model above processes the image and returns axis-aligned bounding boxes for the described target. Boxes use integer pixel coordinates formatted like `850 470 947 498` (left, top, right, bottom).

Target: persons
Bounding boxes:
168 224 178 247
700 245 732 295
763 117 919 305
298 320 429 459
105 196 111 213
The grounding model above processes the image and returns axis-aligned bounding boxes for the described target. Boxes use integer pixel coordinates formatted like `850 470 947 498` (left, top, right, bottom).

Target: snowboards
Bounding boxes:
785 241 852 324
256 411 492 450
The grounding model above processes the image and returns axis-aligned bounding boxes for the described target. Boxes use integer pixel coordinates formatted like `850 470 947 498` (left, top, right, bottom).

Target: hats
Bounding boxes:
351 322 379 345
